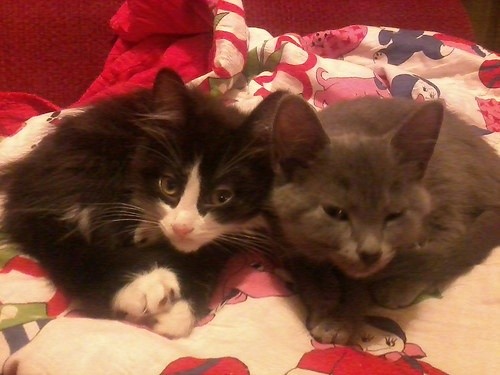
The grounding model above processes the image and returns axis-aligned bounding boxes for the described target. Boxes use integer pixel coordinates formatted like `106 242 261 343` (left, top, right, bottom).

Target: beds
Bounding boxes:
0 0 500 375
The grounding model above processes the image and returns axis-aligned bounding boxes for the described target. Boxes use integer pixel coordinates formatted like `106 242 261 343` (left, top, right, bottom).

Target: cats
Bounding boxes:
0 67 291 341
270 94 499 346
